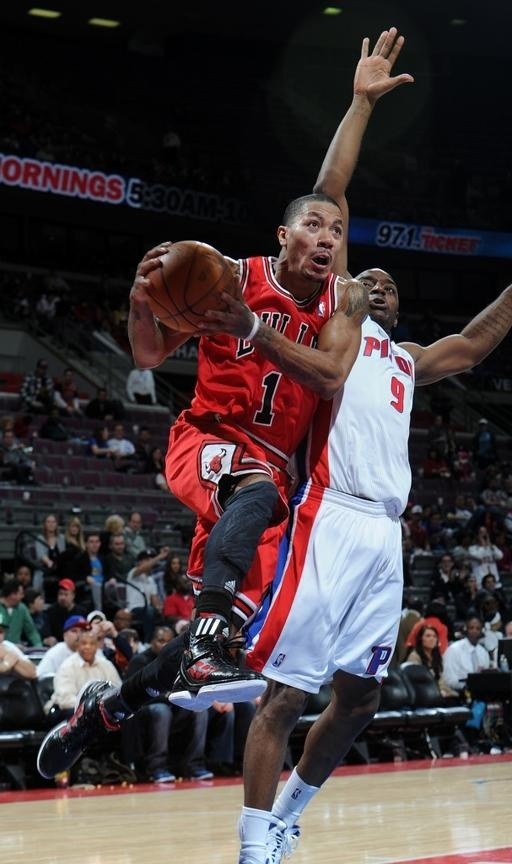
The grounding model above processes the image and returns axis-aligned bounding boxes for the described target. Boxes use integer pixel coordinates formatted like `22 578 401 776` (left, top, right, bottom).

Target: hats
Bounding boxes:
59 579 74 591
137 550 156 560
64 615 92 632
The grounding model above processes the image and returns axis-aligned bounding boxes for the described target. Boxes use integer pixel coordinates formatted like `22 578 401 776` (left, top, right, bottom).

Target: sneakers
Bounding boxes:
186 770 214 780
169 616 268 713
460 752 468 759
490 748 504 755
239 809 300 864
36 681 124 778
147 771 175 783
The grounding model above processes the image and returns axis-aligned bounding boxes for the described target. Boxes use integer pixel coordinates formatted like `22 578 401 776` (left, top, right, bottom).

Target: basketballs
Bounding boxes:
144 240 236 335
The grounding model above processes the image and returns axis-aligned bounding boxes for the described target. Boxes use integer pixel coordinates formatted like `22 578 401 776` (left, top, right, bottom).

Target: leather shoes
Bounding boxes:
210 762 240 777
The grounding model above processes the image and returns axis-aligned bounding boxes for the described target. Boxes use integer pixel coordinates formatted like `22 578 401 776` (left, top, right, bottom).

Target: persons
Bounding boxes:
239 26 512 864
0 195 511 784
